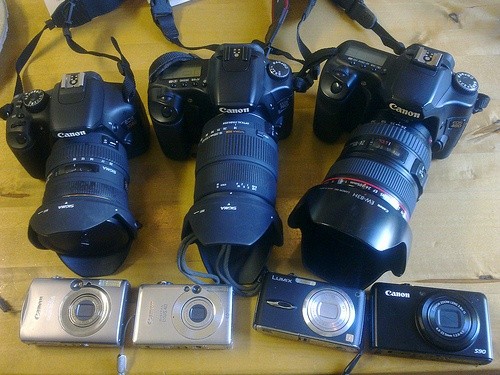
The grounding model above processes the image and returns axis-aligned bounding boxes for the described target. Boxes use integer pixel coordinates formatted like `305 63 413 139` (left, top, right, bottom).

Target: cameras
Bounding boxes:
252 271 367 352
147 43 293 284
287 39 490 290
5 71 148 277
132 280 234 349
19 275 131 346
367 282 493 365
